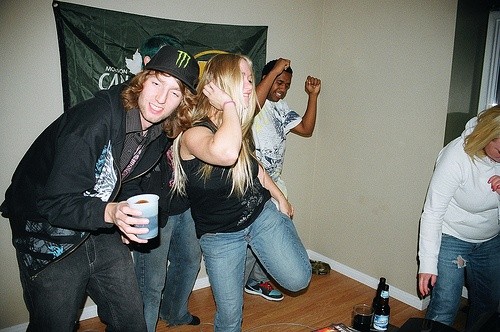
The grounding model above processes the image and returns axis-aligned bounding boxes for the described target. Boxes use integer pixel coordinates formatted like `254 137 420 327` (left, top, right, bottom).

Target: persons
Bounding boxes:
418 103 500 332
0 46 321 332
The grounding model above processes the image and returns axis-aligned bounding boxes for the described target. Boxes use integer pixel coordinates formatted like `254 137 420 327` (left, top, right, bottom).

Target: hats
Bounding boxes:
145 46 200 96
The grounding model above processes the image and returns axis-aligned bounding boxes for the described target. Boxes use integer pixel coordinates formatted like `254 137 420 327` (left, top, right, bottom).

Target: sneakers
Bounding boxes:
243 280 284 301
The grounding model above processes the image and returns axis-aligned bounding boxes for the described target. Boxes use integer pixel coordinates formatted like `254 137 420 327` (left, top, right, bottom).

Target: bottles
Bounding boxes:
372 285 391 332
372 277 386 321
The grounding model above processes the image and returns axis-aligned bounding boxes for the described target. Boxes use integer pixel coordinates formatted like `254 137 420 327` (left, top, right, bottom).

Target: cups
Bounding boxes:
353 304 374 332
127 194 159 241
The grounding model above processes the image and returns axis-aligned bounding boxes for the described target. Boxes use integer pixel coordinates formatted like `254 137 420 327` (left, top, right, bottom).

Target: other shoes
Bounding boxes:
309 259 331 274
